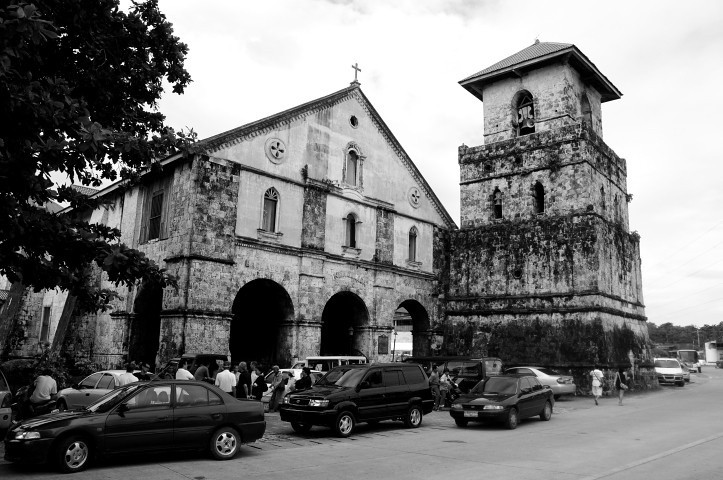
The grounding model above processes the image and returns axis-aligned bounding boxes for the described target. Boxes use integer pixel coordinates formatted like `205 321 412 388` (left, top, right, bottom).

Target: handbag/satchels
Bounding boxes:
620 383 628 390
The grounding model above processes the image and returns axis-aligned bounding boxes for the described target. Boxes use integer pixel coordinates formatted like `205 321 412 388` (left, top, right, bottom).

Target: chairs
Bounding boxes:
144 390 168 406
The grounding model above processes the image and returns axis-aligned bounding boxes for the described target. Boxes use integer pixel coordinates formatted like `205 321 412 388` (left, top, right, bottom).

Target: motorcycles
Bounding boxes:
430 377 464 407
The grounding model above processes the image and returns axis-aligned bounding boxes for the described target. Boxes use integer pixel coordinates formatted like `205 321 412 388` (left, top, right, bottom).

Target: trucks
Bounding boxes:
669 349 700 373
290 355 367 381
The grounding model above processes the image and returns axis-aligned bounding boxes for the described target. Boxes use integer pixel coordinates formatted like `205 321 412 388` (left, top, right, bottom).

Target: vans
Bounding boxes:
402 355 506 395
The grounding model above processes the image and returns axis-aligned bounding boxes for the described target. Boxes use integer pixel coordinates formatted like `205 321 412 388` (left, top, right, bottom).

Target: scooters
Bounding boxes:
9 383 57 421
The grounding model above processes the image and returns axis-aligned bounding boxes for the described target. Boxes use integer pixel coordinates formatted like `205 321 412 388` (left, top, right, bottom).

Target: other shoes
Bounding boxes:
595 400 599 406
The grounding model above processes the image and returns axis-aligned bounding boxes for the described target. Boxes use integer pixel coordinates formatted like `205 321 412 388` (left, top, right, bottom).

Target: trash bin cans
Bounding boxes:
698 367 701 373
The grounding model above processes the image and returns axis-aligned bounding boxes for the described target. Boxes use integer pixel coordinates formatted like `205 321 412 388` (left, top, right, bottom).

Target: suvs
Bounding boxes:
279 362 435 437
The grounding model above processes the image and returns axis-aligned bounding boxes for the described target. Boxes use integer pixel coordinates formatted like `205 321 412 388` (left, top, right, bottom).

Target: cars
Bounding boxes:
4 378 267 473
56 368 190 411
260 368 326 404
0 370 13 431
652 356 691 387
449 373 555 430
501 365 577 402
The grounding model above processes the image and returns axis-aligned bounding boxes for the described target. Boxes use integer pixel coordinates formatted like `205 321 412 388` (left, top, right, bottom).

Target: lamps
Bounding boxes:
348 326 354 336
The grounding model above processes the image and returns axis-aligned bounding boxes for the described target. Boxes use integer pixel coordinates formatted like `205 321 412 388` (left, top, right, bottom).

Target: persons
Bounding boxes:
114 363 140 390
175 358 196 381
614 367 630 405
429 365 458 412
30 369 57 403
696 361 702 373
194 358 210 379
133 362 150 381
214 361 236 396
589 364 604 405
231 360 312 413
